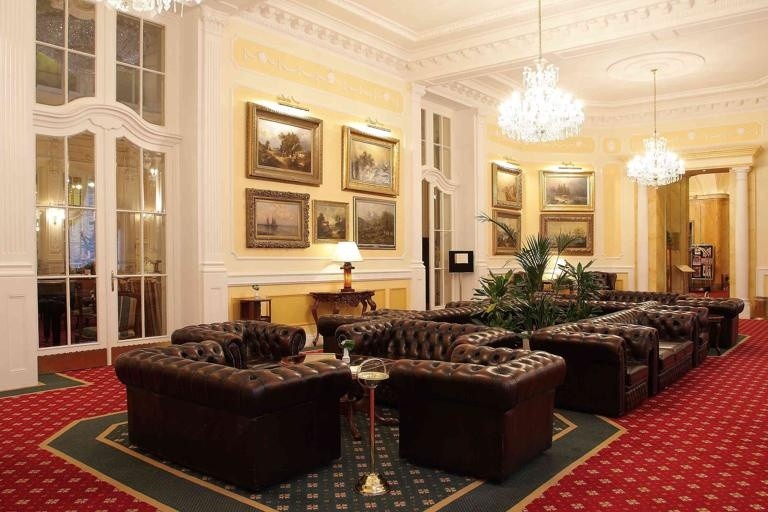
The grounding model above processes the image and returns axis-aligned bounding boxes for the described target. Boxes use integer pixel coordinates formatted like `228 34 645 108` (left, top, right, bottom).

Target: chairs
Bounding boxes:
580 270 617 289
514 271 530 291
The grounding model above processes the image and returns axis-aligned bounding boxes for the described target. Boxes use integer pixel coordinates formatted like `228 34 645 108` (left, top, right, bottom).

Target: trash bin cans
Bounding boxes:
754 297 768 318
722 274 729 290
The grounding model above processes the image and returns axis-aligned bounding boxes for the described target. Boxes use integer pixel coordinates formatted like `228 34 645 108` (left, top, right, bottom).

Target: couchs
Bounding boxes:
318 292 742 419
115 340 351 489
389 344 567 485
171 319 306 368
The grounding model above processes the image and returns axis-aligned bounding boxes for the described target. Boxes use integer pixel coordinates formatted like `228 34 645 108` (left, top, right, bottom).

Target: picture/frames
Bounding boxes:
492 162 522 255
246 101 400 250
539 169 594 256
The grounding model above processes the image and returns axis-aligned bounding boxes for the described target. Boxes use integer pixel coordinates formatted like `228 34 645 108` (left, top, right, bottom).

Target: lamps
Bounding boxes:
498 0 587 142
95 0 201 17
332 241 365 292
627 69 687 187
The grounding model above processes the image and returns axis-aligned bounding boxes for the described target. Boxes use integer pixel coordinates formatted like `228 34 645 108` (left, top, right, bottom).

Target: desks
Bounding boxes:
280 353 398 440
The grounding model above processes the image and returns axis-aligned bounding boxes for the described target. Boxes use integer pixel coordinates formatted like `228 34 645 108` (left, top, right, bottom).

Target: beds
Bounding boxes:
37 256 161 344
